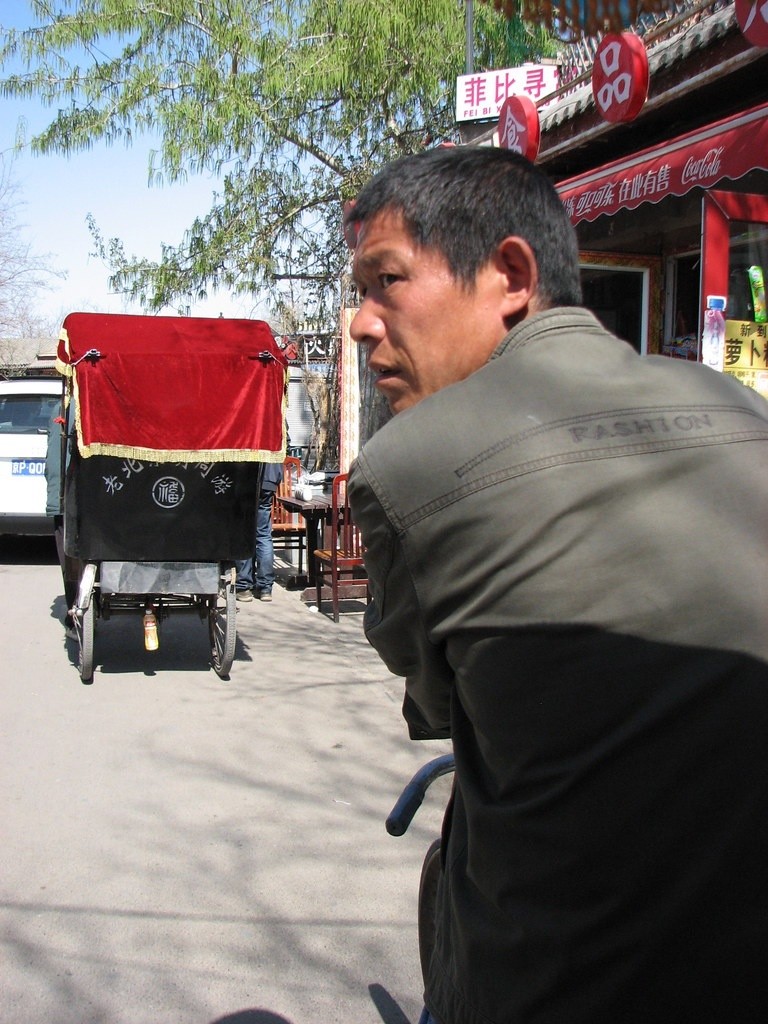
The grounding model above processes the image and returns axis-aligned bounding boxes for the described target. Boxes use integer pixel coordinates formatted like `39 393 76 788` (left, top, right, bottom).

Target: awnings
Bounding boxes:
554 102 768 227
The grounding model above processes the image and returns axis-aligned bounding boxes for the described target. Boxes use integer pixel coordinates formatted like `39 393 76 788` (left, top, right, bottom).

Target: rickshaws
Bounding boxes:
52 310 288 683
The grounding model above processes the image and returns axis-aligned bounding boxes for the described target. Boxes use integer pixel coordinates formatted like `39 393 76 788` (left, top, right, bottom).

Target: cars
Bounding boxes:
0 375 67 538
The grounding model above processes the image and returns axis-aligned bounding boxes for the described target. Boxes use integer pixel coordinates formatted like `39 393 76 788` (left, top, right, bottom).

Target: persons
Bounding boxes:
342 145 768 1024
222 462 285 601
43 378 82 640
